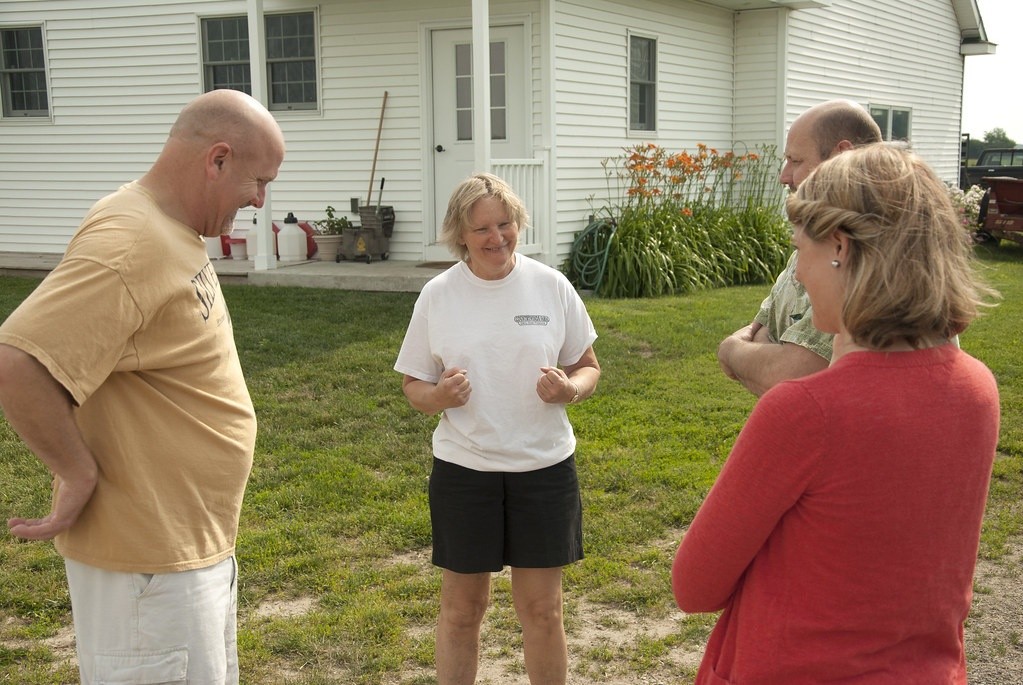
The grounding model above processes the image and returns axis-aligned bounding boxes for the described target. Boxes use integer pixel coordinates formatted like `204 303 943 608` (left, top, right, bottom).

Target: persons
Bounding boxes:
393 173 601 685
672 142 1001 685
718 99 960 400
0 89 285 685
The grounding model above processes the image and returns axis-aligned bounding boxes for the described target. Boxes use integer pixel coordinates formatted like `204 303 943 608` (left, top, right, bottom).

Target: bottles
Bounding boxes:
246 213 277 260
277 213 307 261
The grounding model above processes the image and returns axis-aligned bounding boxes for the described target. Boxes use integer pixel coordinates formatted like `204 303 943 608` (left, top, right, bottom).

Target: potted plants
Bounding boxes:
313 206 348 262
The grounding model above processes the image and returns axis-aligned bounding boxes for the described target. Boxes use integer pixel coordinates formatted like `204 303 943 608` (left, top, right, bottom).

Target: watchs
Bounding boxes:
565 383 579 405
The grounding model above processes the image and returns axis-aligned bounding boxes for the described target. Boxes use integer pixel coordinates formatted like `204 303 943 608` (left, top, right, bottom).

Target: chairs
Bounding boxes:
968 187 999 255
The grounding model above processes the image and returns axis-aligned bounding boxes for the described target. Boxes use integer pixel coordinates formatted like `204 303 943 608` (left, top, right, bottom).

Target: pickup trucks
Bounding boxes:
959 148 1023 190
